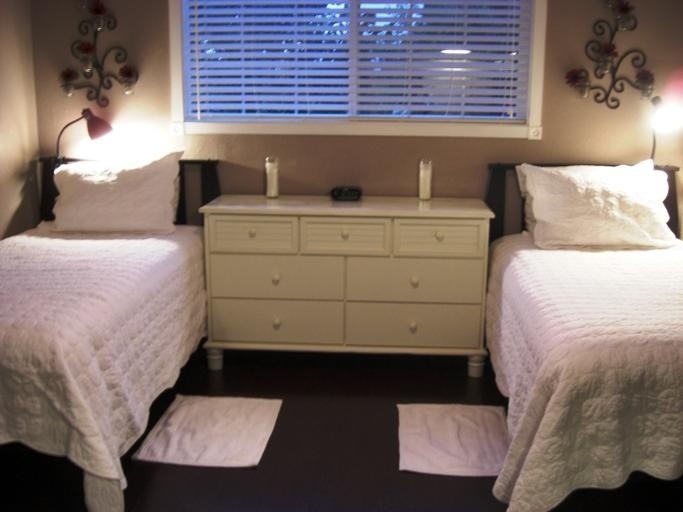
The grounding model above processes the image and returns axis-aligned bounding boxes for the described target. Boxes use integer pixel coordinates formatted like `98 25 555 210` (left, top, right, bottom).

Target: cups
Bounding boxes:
264 156 280 198
417 158 434 200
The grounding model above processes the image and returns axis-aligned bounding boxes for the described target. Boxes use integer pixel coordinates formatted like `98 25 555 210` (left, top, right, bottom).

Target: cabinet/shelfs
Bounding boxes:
204 217 490 379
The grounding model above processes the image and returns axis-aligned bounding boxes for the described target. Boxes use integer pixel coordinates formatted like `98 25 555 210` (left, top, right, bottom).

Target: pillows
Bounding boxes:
515 158 677 250
52 150 184 234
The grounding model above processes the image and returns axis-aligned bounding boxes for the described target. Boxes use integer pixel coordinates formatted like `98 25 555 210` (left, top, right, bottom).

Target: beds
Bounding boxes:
488 161 683 499
1 157 220 512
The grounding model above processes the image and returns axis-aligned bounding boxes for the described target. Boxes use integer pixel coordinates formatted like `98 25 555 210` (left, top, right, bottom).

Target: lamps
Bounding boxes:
53 107 111 162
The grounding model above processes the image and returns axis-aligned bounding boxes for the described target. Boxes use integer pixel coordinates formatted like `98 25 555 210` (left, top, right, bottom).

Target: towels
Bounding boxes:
133 394 283 467
394 400 510 478
